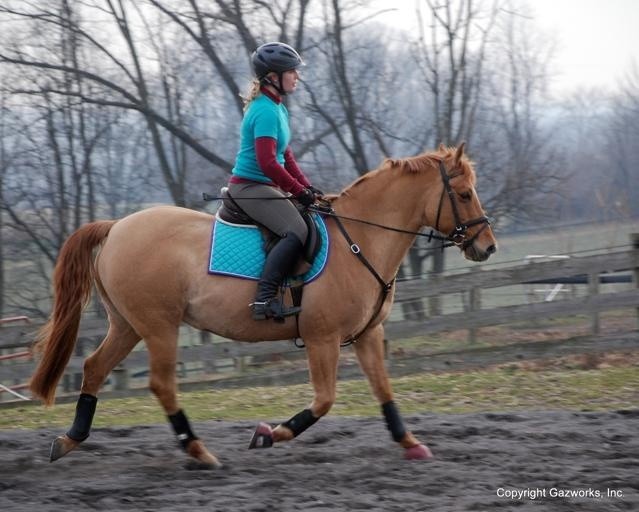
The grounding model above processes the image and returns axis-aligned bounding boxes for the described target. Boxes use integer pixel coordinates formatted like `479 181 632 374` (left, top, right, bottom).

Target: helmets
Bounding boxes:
248 41 306 85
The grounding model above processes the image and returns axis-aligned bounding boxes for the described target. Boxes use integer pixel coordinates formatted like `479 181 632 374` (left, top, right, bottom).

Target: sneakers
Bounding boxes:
247 296 302 323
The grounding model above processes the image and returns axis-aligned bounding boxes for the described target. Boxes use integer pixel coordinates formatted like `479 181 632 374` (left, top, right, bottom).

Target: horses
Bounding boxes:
25 140 499 470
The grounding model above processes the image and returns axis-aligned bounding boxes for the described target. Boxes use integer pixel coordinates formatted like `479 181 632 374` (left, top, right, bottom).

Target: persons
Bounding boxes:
226 41 324 321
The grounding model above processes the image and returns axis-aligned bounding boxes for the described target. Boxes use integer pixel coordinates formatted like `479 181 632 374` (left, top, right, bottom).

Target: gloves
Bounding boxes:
298 189 316 206
309 186 326 197
